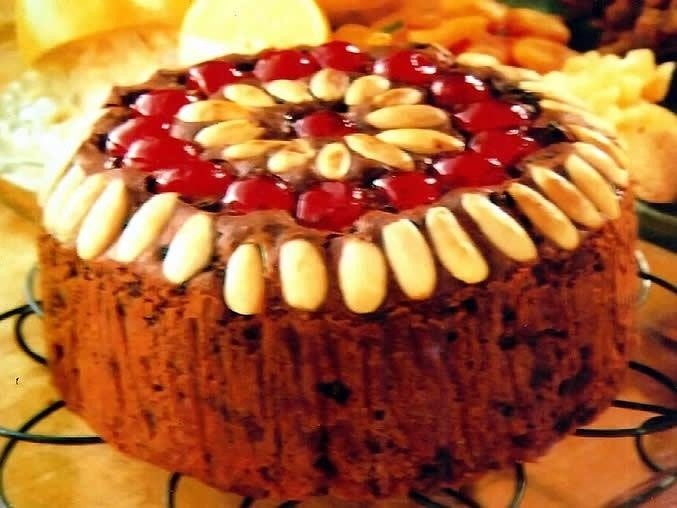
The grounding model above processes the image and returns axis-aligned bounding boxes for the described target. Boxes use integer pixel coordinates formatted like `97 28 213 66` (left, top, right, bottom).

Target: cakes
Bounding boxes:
34 40 640 502
